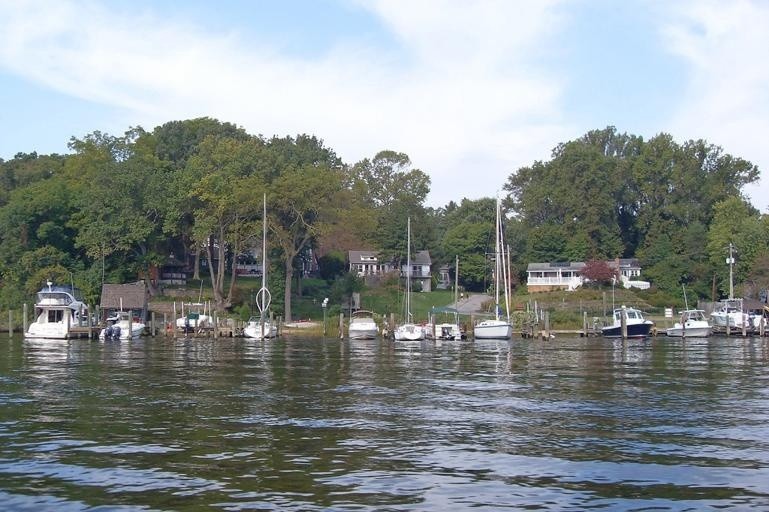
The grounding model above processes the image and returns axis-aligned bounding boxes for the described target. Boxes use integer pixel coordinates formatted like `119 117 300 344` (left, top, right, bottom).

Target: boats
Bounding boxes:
22 278 102 340
95 296 143 340
244 315 279 338
348 309 378 341
423 252 467 342
667 282 711 340
174 313 218 331
603 278 655 338
712 297 768 332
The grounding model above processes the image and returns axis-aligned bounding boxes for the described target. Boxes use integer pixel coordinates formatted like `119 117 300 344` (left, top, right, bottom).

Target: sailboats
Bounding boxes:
472 198 517 339
394 217 427 341
